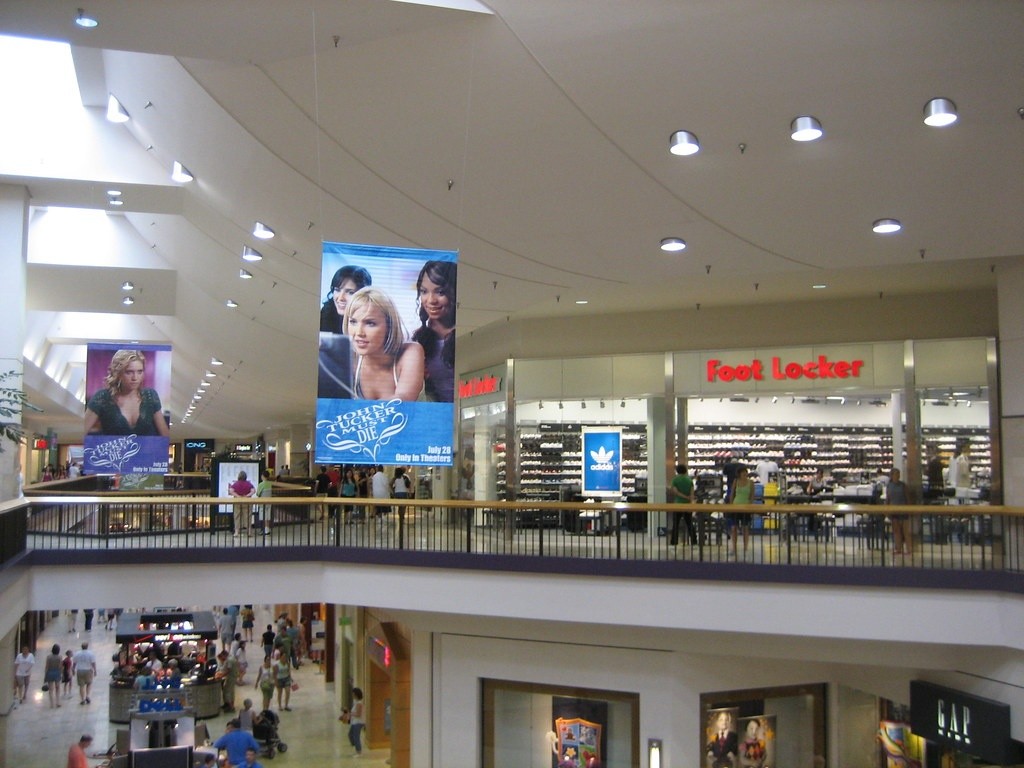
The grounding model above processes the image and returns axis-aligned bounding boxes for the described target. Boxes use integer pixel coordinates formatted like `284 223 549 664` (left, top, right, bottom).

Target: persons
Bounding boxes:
926 443 972 507
723 458 779 490
671 465 698 550
738 718 767 768
67 735 92 768
320 265 372 335
168 463 212 490
84 350 170 437
312 464 433 524
411 261 457 402
806 468 827 494
229 471 256 538
706 711 738 768
256 472 272 536
19 463 83 498
239 697 264 736
877 467 913 555
342 286 426 402
201 719 264 768
15 604 322 711
279 464 290 475
348 687 366 757
730 468 754 555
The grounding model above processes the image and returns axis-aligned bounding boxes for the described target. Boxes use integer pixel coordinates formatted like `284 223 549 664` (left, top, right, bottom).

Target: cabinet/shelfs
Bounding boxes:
494 424 991 526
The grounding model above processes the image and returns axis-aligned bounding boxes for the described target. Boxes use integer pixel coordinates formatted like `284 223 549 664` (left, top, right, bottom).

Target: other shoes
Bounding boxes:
285 707 292 711
279 707 283 711
80 701 85 705
260 532 271 536
86 697 90 703
353 753 362 757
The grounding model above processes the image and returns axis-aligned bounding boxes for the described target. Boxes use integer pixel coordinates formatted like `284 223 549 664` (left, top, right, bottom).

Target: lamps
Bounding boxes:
105 92 274 425
538 386 983 409
661 98 959 252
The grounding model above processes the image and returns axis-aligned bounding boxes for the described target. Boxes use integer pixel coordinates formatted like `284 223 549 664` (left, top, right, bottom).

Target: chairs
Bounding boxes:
764 487 989 549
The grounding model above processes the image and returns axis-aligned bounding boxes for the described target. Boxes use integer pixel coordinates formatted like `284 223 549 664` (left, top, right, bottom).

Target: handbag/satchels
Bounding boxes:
291 683 298 692
402 475 410 487
42 686 49 691
